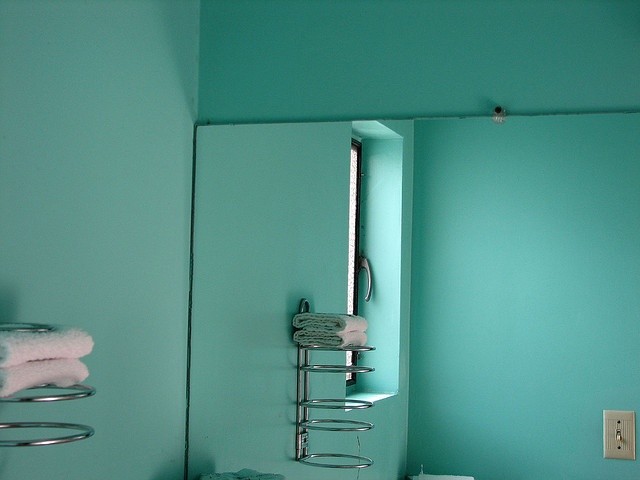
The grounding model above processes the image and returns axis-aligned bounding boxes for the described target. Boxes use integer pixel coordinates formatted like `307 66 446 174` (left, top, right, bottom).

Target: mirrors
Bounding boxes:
191 107 640 480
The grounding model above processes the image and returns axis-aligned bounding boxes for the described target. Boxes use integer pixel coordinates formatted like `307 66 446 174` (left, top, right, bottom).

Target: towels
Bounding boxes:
293 325 368 348
1 325 94 366
0 360 89 398
292 315 369 330
